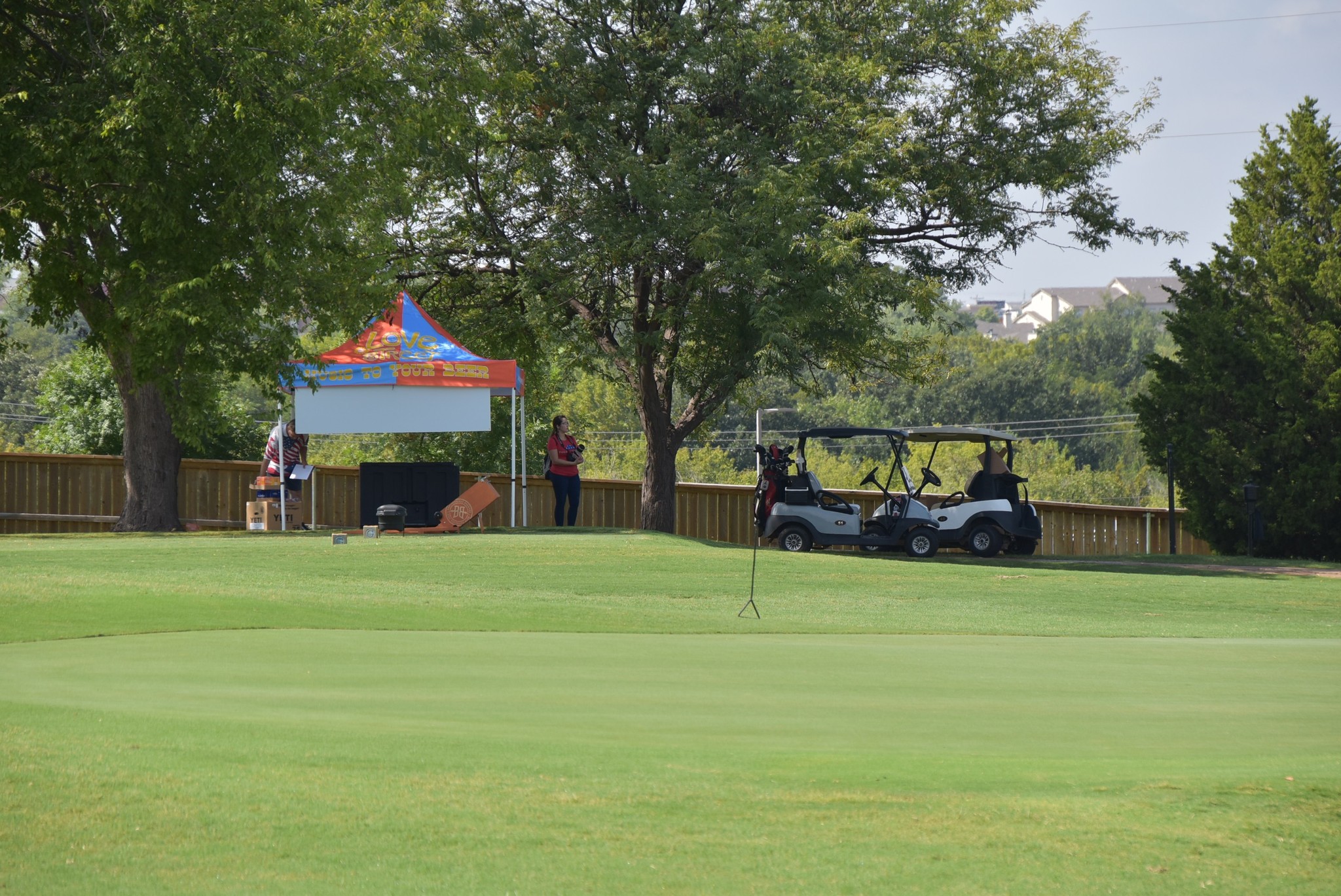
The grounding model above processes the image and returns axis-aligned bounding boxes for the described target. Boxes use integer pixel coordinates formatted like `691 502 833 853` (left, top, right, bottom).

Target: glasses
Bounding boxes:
560 422 569 425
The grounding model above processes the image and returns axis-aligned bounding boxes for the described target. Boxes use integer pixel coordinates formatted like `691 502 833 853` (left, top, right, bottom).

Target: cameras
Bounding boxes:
567 444 585 462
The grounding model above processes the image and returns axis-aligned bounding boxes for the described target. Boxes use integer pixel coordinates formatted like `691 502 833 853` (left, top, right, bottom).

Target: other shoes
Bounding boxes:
302 523 312 531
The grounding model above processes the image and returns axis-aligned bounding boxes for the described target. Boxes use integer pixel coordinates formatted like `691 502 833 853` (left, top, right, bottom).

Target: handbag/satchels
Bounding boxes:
543 437 558 480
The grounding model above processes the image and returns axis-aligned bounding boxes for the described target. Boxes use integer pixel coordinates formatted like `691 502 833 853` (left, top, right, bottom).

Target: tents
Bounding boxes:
276 288 529 528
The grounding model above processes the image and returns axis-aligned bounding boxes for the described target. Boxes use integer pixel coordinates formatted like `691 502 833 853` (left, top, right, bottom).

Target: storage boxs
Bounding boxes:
978 446 1012 474
375 504 407 532
246 476 302 530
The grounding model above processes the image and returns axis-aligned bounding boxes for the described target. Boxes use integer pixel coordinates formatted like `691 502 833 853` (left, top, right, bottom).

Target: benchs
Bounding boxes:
930 470 984 510
807 471 861 515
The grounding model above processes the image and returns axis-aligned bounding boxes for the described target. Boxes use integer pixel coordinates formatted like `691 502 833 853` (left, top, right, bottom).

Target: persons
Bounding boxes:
254 418 310 531
548 415 584 526
